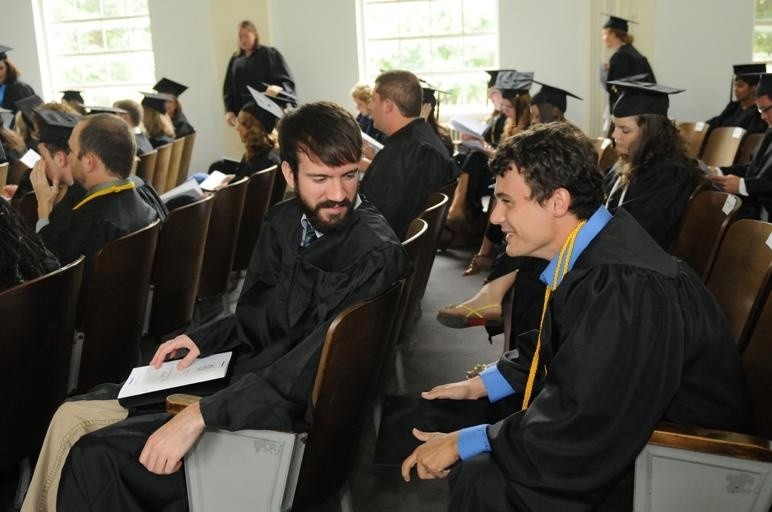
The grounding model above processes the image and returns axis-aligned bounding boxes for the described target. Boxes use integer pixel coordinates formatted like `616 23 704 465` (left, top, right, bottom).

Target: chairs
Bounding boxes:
0 114 772 512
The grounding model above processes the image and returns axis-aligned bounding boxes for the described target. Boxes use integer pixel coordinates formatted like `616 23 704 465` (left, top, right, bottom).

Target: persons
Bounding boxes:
15 101 417 511
403 123 756 512
220 17 294 127
2 48 772 378
596 14 658 108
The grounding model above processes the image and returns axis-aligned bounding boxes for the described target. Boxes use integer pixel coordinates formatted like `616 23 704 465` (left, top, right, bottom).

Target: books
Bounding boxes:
112 348 232 409
373 388 494 475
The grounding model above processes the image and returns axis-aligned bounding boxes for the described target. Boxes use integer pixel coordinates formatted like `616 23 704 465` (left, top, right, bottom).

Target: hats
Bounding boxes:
720 63 772 103
1 76 188 143
245 82 297 123
601 11 639 33
602 74 687 118
419 81 453 124
486 70 583 112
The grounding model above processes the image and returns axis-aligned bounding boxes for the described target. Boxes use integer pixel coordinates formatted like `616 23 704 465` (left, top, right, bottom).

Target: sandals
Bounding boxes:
462 255 495 276
437 299 505 329
